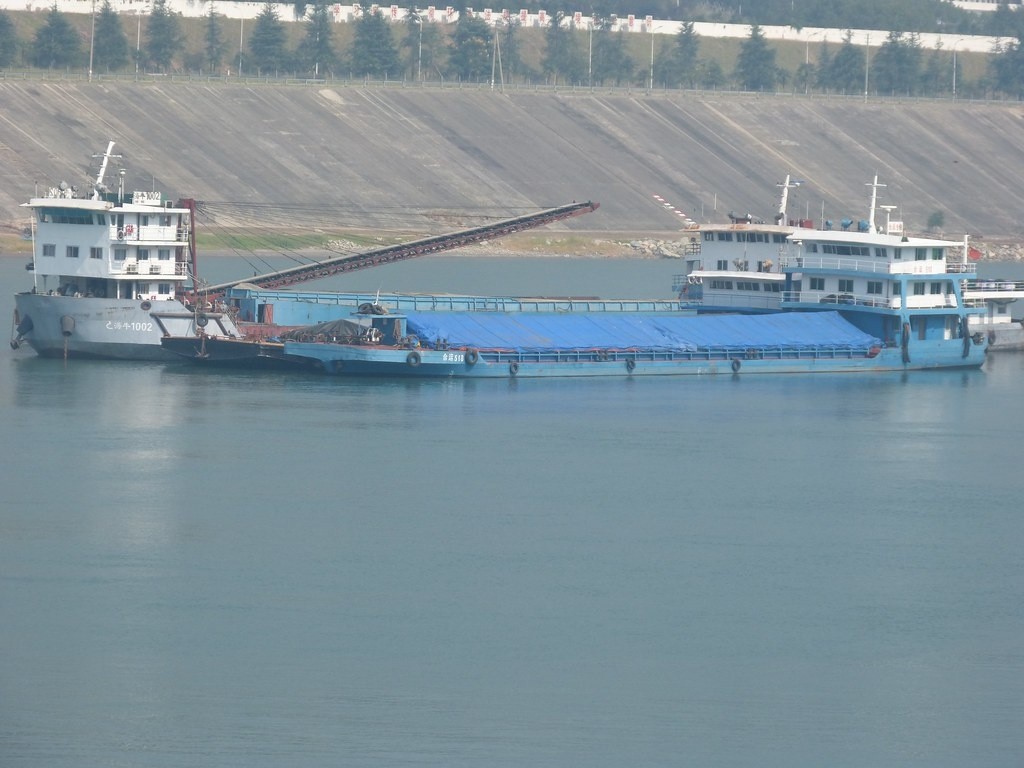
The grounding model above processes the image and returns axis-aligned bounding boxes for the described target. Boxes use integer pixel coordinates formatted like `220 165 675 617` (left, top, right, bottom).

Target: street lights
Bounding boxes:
804 31 820 94
953 38 964 94
650 24 664 88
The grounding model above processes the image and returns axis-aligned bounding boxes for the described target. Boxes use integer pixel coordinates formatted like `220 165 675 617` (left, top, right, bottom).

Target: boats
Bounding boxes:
4 130 1024 378
150 259 994 379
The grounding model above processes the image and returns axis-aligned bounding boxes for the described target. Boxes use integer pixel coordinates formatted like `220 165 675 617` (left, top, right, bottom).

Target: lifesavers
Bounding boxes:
117 230 124 241
197 313 209 327
627 360 636 369
732 360 741 372
14 309 18 324
407 352 421 368
140 301 151 311
988 333 996 345
510 363 519 374
464 350 478 365
126 224 133 234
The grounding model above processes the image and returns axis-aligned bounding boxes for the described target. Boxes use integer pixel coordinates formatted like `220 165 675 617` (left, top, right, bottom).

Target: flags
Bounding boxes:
969 247 985 260
